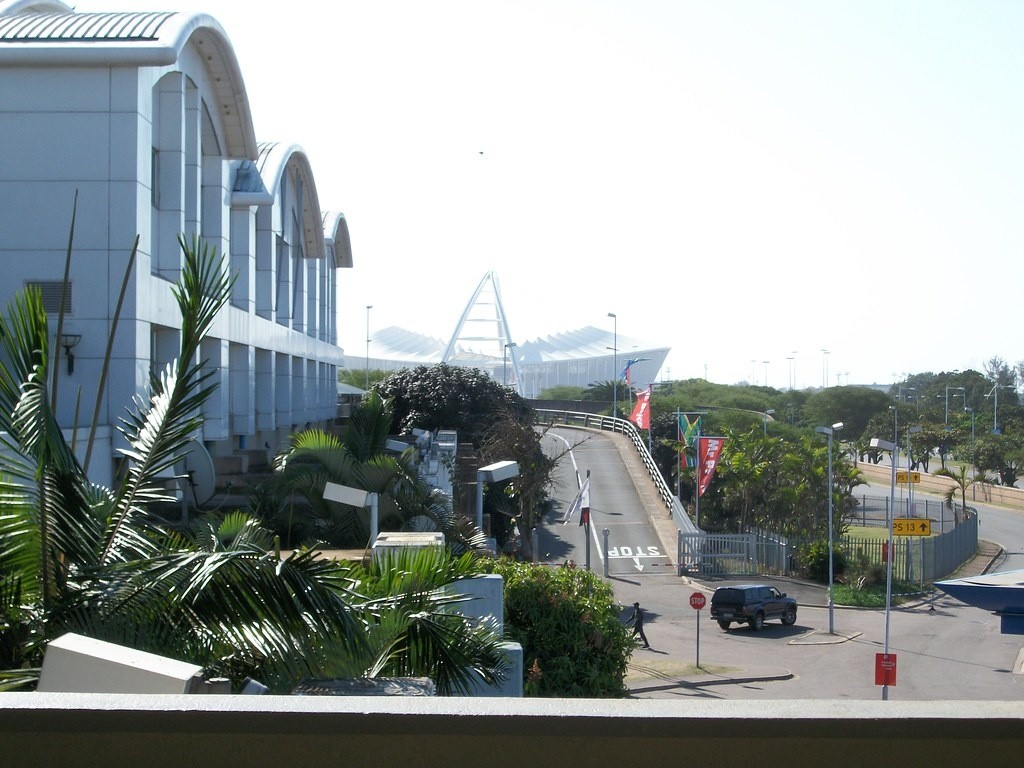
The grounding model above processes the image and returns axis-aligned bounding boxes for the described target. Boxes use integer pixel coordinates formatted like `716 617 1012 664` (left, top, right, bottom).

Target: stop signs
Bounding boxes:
690 591 706 610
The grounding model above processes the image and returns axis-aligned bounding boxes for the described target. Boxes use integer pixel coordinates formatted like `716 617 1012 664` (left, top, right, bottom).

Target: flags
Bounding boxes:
679 413 703 469
630 387 652 429
698 439 724 498
563 476 591 528
620 360 638 384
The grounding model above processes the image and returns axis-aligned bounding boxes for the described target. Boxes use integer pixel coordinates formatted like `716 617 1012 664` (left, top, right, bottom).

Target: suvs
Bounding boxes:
710 584 798 632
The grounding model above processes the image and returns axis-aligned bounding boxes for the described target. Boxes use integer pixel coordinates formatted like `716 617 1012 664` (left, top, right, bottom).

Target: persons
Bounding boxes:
624 602 649 648
771 591 775 598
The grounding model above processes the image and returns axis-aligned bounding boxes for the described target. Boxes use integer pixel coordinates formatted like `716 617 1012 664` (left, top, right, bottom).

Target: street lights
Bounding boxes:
814 426 834 634
322 481 379 552
964 406 975 501
889 405 898 446
907 424 924 583
608 312 617 417
503 342 518 386
869 437 898 700
916 395 927 413
475 461 520 551
945 384 965 424
898 385 916 402
994 385 1017 431
750 347 831 391
764 409 777 435
366 305 373 391
938 392 966 413
